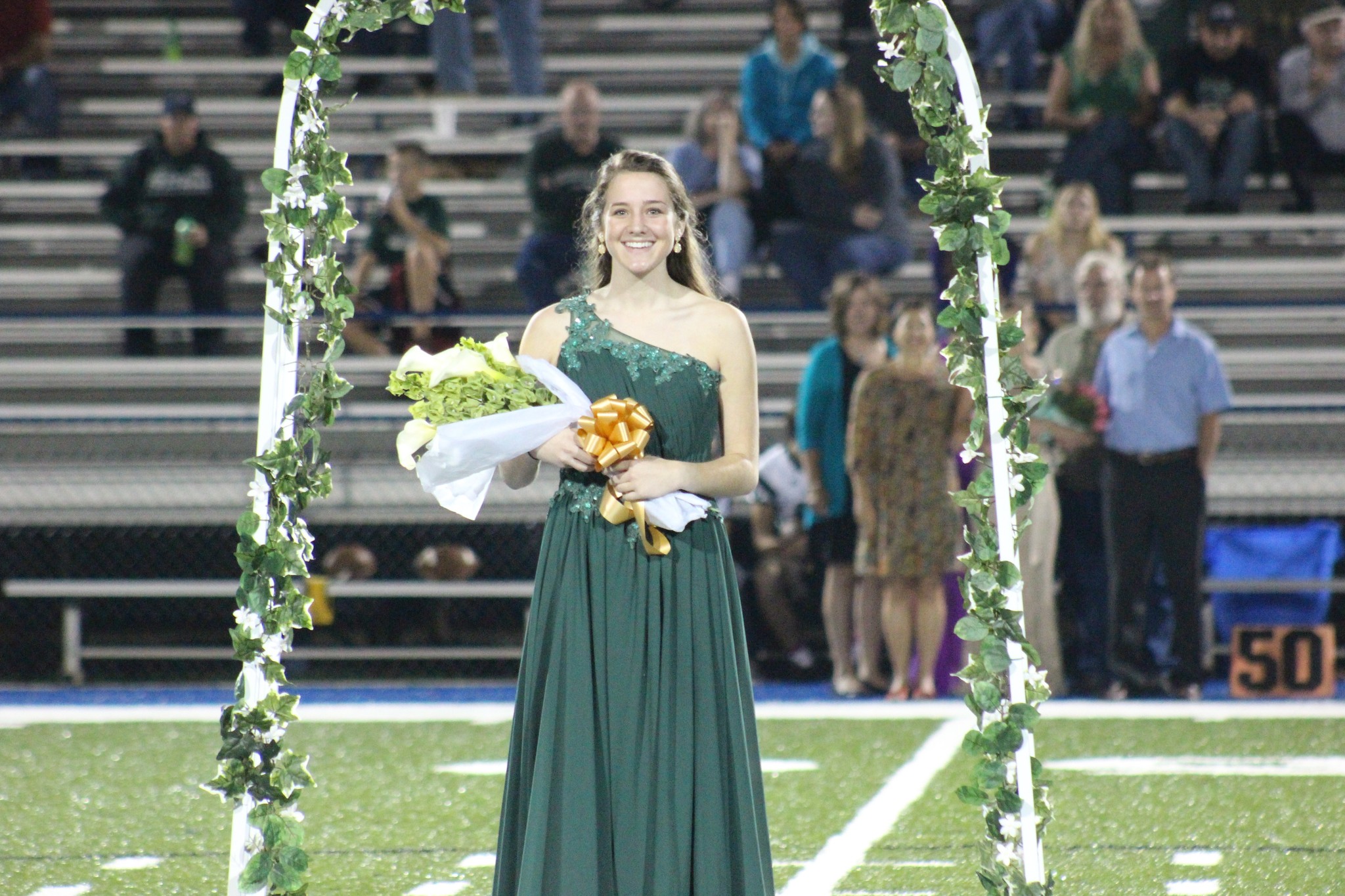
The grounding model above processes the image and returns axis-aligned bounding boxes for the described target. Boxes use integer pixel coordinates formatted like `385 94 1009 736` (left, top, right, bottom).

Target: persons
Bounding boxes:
0 0 1345 699
490 153 776 896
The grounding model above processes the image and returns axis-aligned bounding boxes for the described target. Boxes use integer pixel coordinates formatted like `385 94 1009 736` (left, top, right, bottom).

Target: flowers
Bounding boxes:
1023 382 1110 444
385 332 711 555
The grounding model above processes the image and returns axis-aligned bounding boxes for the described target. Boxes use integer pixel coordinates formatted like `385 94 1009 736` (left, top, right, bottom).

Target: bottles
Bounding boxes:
172 216 195 265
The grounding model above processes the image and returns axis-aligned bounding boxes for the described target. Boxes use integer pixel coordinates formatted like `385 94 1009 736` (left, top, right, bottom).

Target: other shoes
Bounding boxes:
1106 681 1127 700
913 680 935 700
1178 682 1202 701
888 677 911 701
859 672 885 689
834 673 862 698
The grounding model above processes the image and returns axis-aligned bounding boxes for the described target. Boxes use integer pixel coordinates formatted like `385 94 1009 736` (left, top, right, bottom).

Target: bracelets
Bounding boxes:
527 451 540 460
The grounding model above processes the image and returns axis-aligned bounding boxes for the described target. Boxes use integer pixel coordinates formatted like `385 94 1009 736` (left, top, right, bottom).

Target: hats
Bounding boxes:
1203 5 1245 28
166 91 193 112
